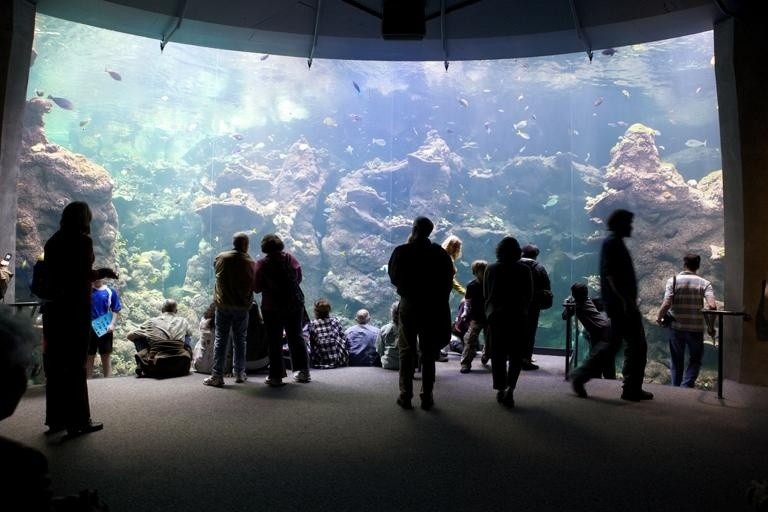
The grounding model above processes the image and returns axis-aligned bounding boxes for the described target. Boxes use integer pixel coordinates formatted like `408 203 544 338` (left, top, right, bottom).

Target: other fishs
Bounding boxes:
15 10 726 350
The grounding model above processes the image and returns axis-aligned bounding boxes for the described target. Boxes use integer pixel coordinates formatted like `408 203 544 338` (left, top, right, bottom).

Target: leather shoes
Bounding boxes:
68 420 103 435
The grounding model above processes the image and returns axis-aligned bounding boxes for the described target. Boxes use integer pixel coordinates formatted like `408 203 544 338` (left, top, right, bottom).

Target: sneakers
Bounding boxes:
202 371 310 385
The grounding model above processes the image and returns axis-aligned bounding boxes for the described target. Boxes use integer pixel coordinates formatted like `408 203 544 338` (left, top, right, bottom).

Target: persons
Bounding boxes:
375 300 419 370
88 267 120 381
483 237 550 404
436 235 487 373
345 308 382 367
203 232 255 385
567 209 653 400
192 300 270 373
43 199 119 441
129 299 192 378
656 253 717 388
303 298 349 369
389 217 456 408
561 282 616 379
0 299 48 510
254 234 311 383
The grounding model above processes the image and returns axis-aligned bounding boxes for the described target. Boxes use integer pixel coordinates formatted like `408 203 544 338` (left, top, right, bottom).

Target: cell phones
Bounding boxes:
1 253 12 266
